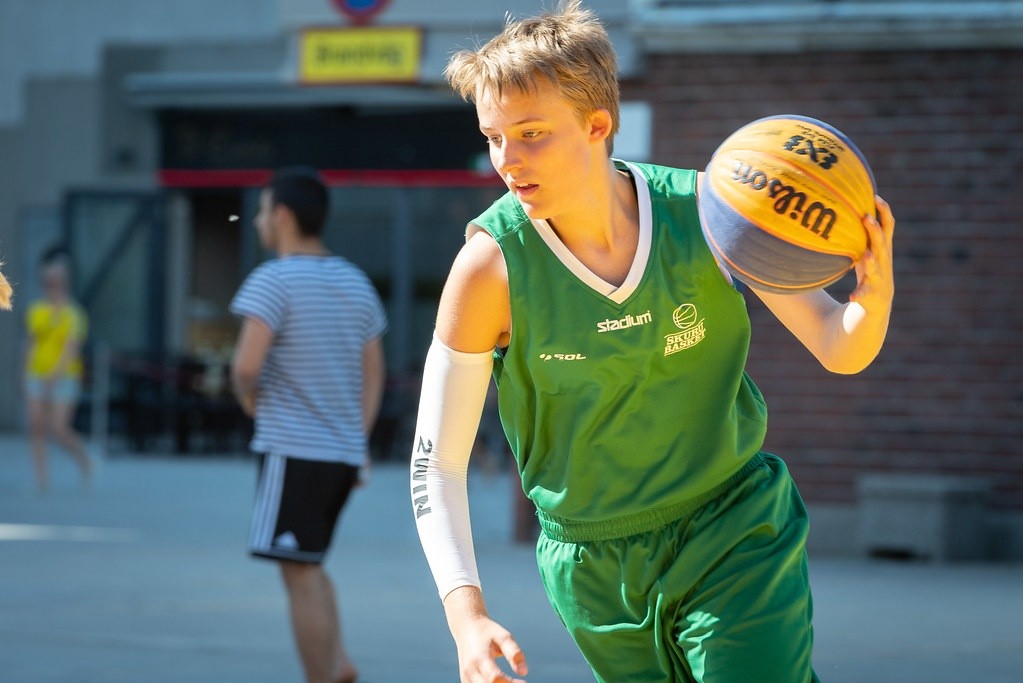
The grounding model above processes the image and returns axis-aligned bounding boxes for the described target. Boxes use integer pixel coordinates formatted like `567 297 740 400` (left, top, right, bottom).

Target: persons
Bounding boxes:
230 165 393 683
411 1 894 683
24 251 99 497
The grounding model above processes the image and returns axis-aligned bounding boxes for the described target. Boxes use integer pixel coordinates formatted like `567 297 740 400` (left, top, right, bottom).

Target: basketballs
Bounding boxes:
697 113 878 297
